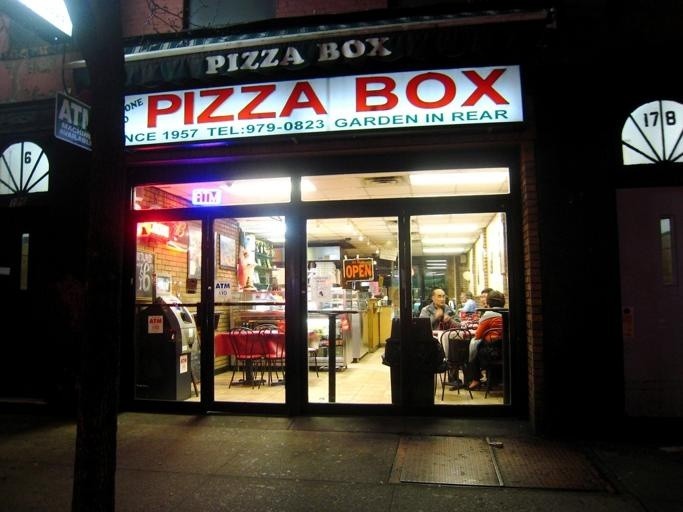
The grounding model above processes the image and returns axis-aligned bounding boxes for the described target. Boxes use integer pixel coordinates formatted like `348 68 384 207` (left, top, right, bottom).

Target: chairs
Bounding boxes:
228 324 319 388
439 328 504 401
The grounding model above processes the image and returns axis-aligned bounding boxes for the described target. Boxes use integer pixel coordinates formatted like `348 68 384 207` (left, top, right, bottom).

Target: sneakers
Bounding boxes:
447 378 482 390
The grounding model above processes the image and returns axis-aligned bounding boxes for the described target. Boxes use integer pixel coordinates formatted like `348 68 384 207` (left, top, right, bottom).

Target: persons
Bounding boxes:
462 290 506 390
454 291 475 319
416 287 469 386
479 288 492 308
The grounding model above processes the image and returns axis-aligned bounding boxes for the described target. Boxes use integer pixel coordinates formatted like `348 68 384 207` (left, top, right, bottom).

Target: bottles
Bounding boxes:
253 240 276 255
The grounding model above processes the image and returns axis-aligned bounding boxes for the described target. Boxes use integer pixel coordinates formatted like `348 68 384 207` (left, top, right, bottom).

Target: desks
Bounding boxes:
431 329 477 393
214 331 312 389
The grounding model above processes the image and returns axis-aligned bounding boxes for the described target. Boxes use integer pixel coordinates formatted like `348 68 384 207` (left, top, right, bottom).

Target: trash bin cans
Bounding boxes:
136 295 199 401
382 318 448 407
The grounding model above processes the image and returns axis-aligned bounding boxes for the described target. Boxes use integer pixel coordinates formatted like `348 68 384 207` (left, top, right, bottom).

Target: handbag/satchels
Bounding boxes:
445 328 471 363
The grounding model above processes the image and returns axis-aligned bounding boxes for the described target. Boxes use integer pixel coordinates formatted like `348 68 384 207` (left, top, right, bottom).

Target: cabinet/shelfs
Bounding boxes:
252 239 275 287
